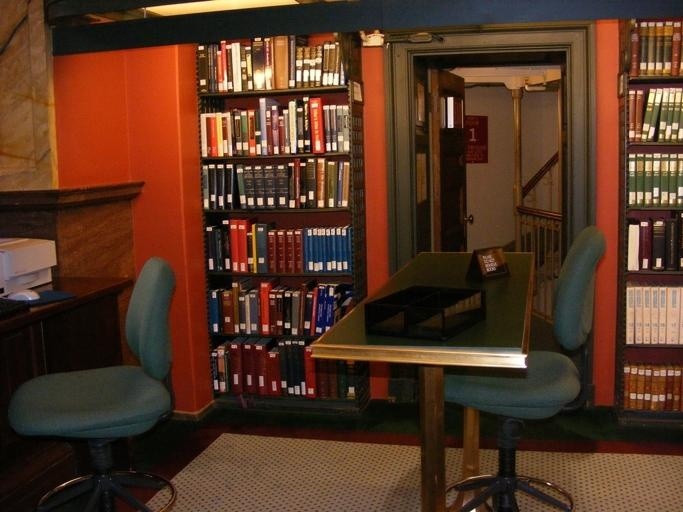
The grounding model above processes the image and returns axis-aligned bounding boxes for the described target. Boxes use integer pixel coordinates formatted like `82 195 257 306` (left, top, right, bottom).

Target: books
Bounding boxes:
197 33 347 95
625 17 683 413
210 337 356 400
208 278 356 338
205 216 353 274
200 96 350 158
202 158 349 209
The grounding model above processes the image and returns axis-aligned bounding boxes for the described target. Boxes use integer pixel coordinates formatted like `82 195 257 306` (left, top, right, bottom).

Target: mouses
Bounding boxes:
8 289 40 301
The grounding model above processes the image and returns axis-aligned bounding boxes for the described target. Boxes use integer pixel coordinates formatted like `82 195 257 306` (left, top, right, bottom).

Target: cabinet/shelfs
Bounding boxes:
196 30 370 431
614 16 683 432
0 277 133 512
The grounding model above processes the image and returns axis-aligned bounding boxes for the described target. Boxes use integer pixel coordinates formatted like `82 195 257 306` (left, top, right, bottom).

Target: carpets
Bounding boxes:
139 432 683 512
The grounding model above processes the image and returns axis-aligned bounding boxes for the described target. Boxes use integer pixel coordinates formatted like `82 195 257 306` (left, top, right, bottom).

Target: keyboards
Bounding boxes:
0 297 31 322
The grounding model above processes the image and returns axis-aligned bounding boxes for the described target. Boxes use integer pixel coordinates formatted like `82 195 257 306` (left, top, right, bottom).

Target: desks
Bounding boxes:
311 252 536 511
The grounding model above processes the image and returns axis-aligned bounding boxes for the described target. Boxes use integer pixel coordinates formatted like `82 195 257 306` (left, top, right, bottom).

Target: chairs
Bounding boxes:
443 225 607 512
7 256 178 512
